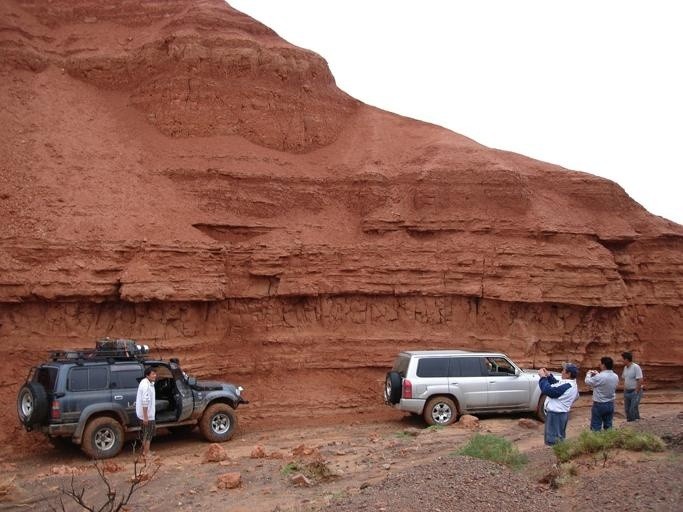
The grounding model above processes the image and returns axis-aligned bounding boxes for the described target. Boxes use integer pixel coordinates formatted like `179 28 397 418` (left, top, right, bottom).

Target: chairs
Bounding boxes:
154 399 170 413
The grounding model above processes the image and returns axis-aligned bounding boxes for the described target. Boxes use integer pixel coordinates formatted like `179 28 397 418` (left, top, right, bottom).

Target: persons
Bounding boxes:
618 352 646 420
536 361 579 447
584 357 620 431
134 366 157 460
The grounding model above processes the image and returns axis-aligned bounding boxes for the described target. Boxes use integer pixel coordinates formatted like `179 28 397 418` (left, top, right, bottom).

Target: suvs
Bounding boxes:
16 338 247 459
382 348 579 426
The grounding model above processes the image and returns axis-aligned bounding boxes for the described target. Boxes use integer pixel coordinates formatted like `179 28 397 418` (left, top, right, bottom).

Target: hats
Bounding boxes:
562 362 578 376
